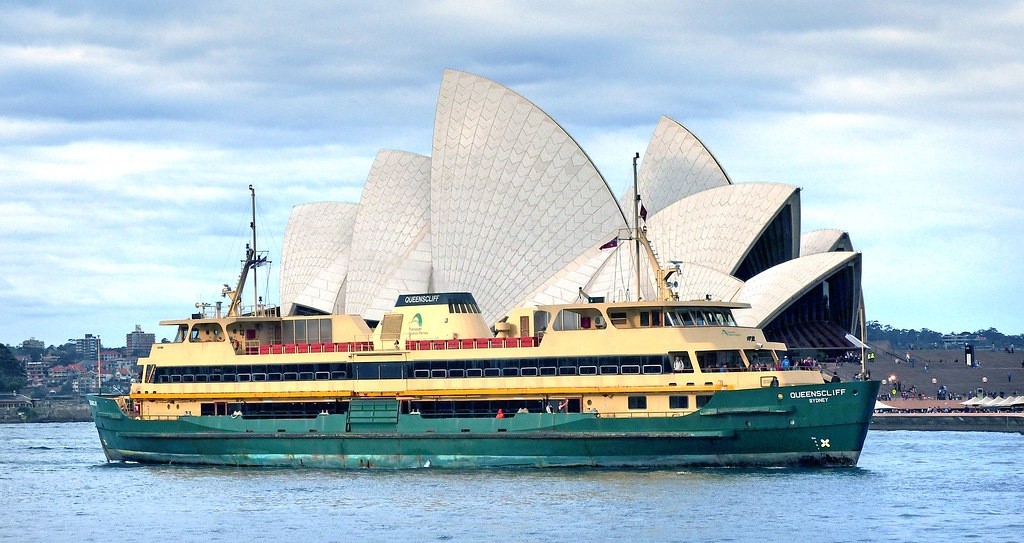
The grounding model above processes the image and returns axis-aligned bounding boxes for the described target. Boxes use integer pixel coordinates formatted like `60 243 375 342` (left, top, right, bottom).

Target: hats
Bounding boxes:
784 356 787 358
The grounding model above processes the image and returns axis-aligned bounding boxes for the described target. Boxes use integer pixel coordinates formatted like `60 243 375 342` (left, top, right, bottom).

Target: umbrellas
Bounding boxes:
959 395 1024 406
874 400 897 410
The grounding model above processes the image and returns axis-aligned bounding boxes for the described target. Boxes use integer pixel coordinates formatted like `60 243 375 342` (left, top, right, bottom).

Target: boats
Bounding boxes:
83 151 881 473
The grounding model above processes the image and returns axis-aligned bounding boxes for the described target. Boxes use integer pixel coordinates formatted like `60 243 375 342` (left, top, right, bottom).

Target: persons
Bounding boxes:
836 350 874 365
496 409 504 418
748 362 767 371
558 400 567 414
518 405 529 413
906 352 911 363
1010 343 1014 353
874 378 973 414
831 372 841 383
546 402 555 414
1008 372 1011 382
234 407 243 416
707 363 728 372
777 355 821 371
673 356 684 373
770 377 779 387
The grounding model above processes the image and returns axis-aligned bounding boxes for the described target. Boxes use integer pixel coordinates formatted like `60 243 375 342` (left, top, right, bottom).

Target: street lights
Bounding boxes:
982 377 987 396
882 380 887 400
932 378 937 400
